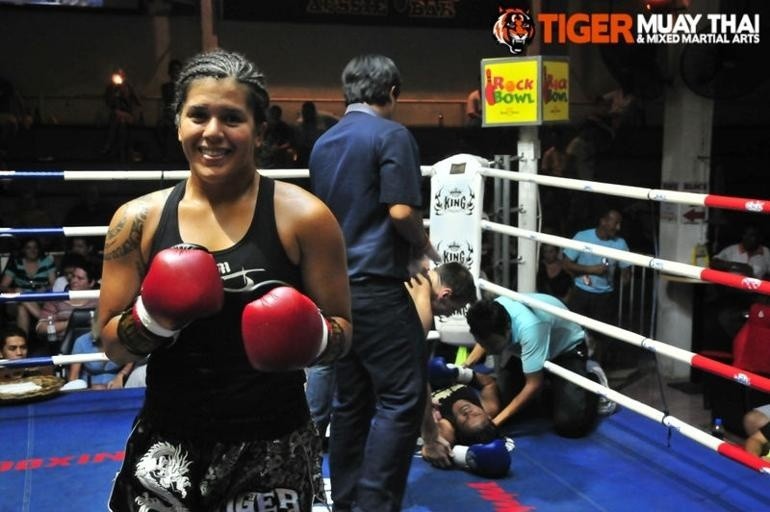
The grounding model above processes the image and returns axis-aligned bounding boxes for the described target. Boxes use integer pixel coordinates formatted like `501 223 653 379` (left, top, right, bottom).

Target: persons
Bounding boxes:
0 45 770 512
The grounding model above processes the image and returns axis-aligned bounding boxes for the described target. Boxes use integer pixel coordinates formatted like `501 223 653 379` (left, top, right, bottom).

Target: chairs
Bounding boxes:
53 309 96 381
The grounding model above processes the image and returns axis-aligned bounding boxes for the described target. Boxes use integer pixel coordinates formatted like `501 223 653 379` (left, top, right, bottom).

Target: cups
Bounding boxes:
598 256 609 277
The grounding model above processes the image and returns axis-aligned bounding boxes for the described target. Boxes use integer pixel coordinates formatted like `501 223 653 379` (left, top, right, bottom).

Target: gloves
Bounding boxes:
132 244 223 338
452 440 515 479
241 286 333 372
426 357 474 386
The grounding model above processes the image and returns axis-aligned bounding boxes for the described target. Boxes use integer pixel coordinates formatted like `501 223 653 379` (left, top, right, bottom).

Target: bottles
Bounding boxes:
710 418 725 442
54 353 66 380
45 316 57 342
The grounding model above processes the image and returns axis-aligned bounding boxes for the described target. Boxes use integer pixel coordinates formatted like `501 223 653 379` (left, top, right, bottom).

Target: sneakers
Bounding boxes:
587 360 616 416
